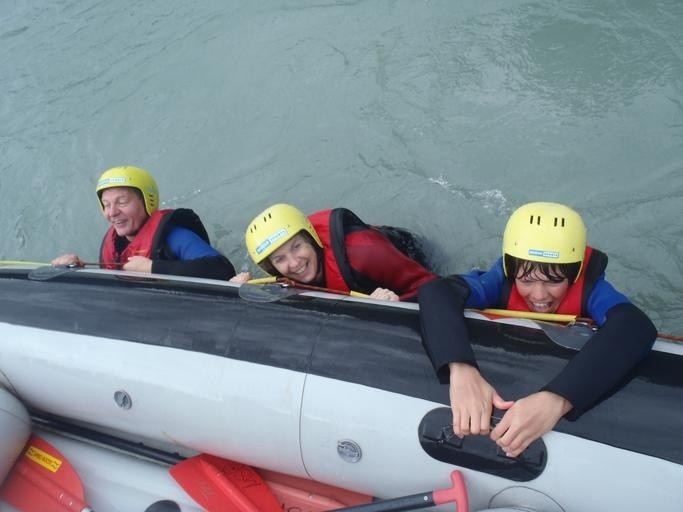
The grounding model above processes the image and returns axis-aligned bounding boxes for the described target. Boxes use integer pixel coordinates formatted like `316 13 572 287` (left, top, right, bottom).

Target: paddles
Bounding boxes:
29 414 470 512
0 433 94 512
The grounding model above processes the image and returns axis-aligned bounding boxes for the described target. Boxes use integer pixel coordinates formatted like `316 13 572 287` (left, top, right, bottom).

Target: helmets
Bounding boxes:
245 203 324 278
94 166 159 217
501 202 586 283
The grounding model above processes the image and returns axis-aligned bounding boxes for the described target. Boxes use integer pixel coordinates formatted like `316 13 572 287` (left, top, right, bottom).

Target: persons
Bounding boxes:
229 203 438 309
415 201 660 460
48 167 236 282
